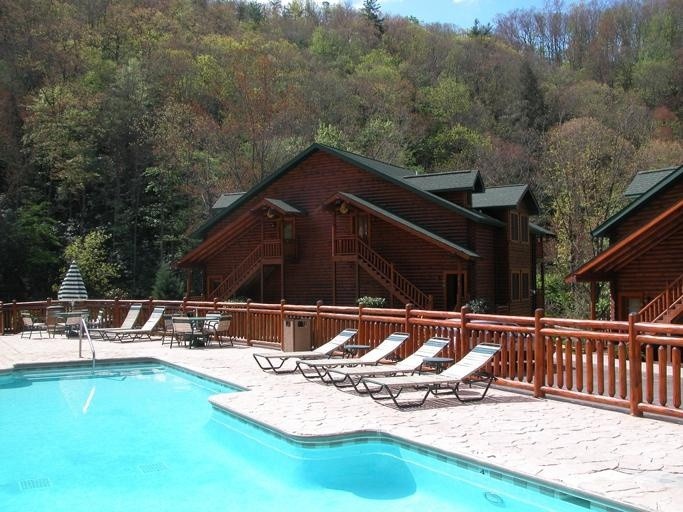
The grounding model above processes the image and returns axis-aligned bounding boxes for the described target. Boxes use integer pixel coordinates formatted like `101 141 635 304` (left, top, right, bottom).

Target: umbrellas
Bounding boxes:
57 259 88 311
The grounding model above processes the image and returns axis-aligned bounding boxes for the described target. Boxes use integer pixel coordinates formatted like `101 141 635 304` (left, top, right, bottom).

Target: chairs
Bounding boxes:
252 328 503 409
19 303 233 350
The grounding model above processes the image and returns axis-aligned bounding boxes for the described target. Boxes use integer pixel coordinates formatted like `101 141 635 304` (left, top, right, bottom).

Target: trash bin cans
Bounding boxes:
46 306 65 326
284 319 311 351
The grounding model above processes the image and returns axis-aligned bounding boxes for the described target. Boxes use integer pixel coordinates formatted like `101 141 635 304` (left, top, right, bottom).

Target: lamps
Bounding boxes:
340 203 348 214
266 206 275 219
